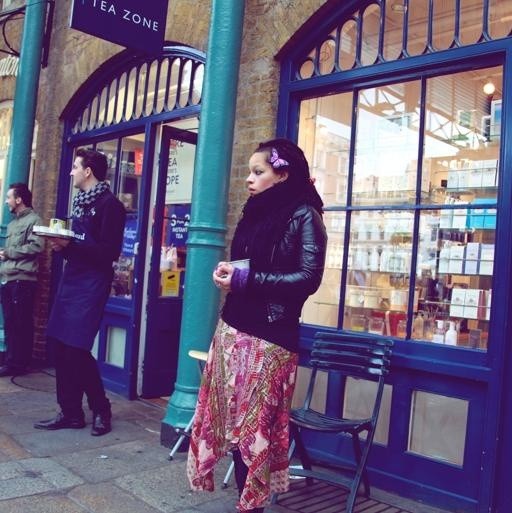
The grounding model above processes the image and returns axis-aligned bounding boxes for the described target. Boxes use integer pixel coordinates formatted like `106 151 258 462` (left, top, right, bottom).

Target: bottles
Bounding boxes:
349 314 407 338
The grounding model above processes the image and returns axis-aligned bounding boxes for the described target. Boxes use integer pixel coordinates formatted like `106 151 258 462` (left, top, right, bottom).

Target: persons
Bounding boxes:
0 184 45 377
33 148 126 436
186 140 328 513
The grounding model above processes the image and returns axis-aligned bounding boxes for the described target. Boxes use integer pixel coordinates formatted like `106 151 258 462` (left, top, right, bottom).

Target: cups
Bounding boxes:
33 217 75 237
217 258 251 269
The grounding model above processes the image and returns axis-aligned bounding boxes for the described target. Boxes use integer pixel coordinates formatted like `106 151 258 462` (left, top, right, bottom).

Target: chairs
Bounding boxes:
269 328 395 513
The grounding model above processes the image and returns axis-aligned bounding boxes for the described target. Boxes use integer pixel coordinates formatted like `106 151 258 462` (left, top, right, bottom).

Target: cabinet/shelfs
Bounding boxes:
105 168 141 212
313 183 499 321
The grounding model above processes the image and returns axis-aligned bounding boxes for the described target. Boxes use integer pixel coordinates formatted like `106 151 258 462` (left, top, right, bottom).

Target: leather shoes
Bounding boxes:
0 364 28 376
32 411 85 430
91 410 112 436
